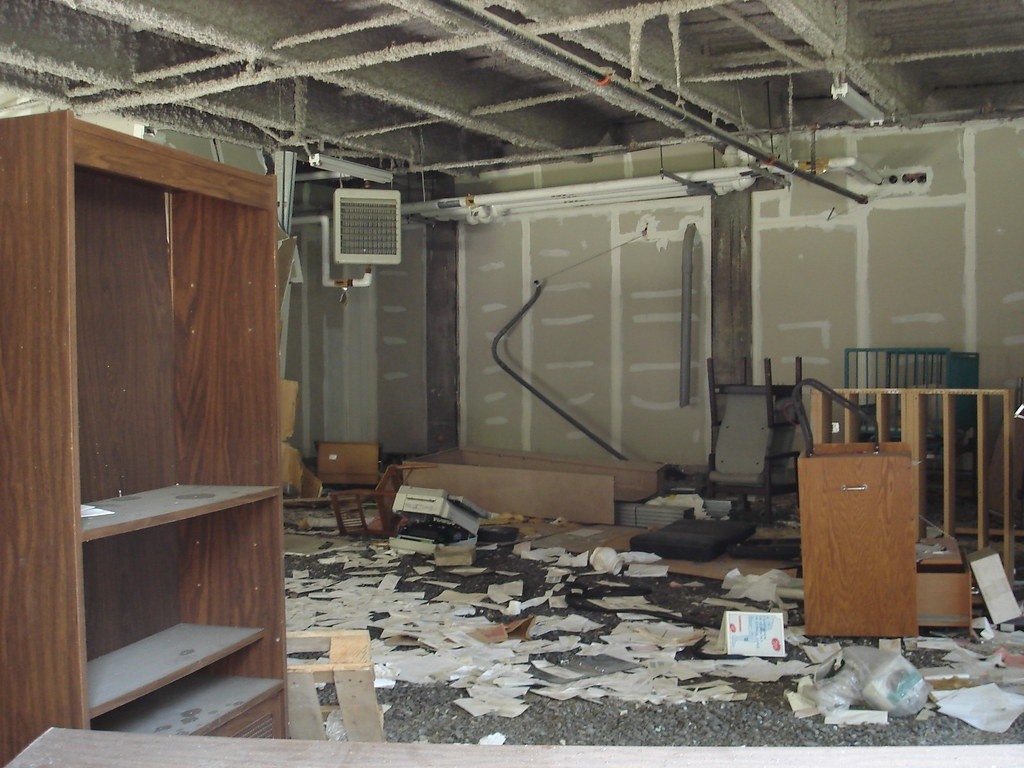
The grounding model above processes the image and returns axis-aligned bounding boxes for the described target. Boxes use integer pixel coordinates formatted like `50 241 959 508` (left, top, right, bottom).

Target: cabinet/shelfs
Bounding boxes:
0 106 286 768
402 449 670 524
797 440 974 638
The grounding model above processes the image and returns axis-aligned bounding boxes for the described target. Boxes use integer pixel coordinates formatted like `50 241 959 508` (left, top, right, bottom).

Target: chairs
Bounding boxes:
328 464 438 539
705 354 814 517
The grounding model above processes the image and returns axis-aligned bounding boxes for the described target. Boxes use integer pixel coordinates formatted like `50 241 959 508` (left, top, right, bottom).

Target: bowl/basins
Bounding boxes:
477 525 519 542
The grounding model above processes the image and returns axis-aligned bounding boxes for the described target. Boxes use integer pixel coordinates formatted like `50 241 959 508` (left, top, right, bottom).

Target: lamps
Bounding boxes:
831 81 885 127
309 153 393 184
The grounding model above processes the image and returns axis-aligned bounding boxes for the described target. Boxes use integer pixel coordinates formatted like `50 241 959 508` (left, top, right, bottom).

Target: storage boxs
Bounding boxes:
968 547 1022 626
315 440 382 484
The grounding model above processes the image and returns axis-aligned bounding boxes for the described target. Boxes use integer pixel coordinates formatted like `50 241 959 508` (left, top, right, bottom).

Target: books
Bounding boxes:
717 610 785 657
